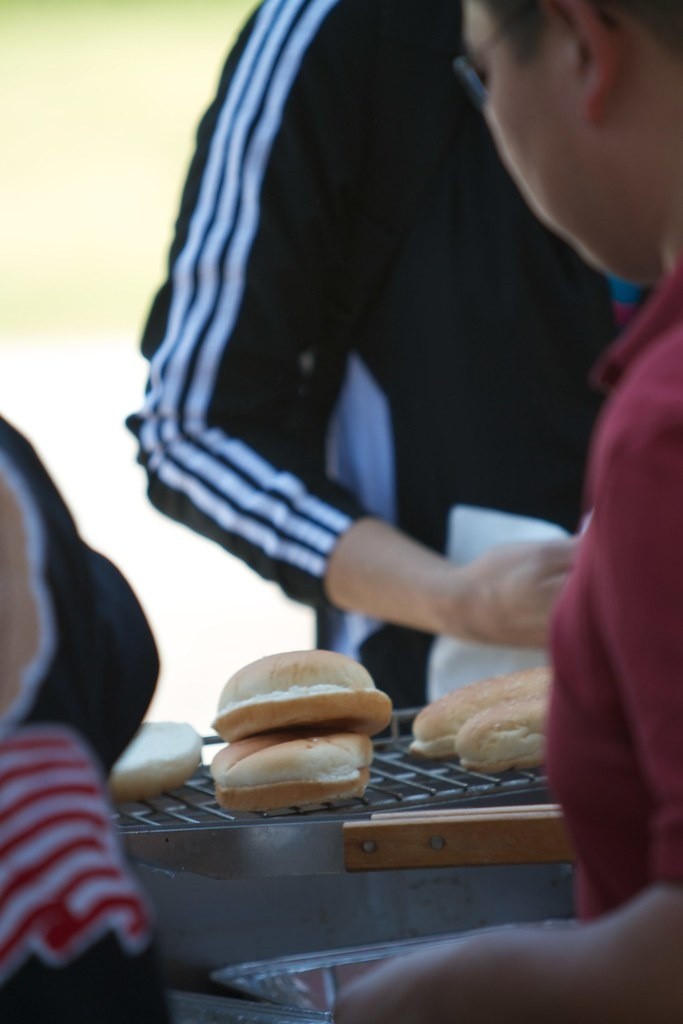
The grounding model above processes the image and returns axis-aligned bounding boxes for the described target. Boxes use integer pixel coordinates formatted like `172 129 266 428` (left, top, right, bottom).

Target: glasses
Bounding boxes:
452 7 528 104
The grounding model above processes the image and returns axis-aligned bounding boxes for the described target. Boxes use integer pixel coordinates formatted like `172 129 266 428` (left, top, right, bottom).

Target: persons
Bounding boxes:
329 0 683 1024
125 1 643 703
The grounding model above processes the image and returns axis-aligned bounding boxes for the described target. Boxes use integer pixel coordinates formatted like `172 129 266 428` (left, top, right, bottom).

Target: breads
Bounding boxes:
106 650 551 812
